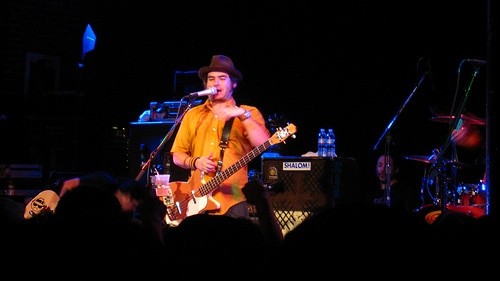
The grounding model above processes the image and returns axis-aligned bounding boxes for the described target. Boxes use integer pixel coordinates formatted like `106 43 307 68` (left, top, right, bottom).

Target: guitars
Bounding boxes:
162 123 297 223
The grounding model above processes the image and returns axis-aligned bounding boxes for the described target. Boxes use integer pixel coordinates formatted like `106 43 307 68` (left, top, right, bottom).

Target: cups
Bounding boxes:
156 174 170 197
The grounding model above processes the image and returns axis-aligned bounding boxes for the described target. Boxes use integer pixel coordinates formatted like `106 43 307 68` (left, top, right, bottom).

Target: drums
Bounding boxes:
444 181 487 218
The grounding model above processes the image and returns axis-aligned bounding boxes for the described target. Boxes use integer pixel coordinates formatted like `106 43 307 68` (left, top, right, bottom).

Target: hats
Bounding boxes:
198 55 243 83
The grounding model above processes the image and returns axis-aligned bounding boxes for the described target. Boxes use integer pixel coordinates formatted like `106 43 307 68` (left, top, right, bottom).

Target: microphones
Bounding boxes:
431 114 463 123
465 59 486 67
185 86 218 99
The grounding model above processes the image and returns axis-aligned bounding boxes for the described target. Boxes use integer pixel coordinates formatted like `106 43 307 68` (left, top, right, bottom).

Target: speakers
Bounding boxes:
261 156 348 212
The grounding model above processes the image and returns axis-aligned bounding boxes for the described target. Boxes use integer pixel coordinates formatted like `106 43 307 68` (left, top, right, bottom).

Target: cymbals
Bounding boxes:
431 115 484 126
404 153 465 168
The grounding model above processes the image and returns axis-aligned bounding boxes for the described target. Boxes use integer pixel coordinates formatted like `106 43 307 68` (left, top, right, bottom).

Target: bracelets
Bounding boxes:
238 110 251 122
190 156 200 169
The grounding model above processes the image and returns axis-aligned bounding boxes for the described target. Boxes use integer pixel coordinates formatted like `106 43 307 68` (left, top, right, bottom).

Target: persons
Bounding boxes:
170 54 271 219
0 207 500 281
56 175 145 220
370 152 411 211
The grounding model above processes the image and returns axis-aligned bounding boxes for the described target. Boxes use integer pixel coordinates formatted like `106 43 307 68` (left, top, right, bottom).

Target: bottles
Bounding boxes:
318 129 327 157
8 185 14 195
327 129 335 157
